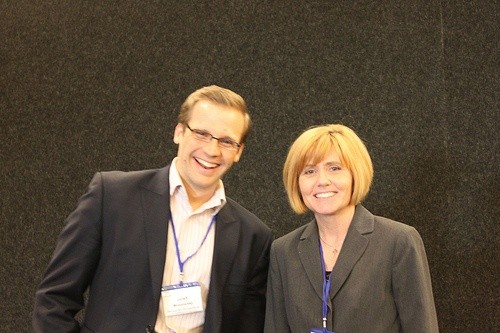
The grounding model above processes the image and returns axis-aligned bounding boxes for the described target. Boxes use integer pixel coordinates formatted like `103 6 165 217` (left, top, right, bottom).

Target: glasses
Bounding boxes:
181 119 243 153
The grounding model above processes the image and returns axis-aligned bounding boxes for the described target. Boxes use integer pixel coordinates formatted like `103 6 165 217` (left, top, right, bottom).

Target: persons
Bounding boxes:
263 125 440 333
29 84 273 333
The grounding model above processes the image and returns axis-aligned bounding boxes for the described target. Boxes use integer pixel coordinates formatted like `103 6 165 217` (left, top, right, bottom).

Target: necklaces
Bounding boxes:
319 234 345 256
